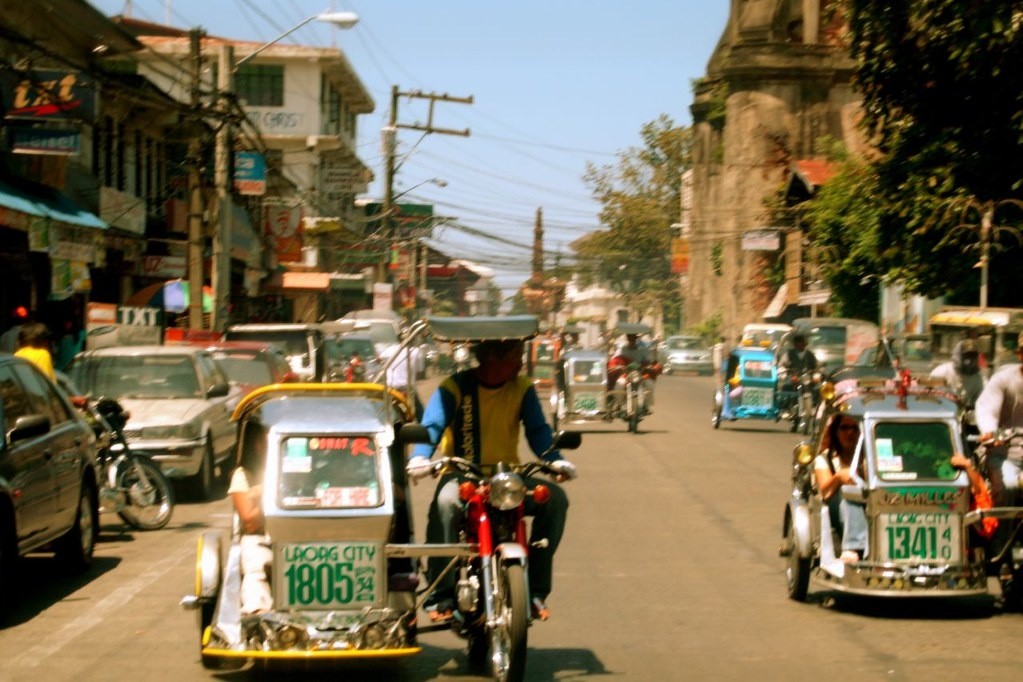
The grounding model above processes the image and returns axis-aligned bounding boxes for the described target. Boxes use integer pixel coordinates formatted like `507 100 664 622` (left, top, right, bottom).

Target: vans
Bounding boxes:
792 317 879 373
734 323 791 370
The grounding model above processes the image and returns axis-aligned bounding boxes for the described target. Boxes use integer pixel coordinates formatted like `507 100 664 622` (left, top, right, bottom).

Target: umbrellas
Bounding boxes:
118 277 232 343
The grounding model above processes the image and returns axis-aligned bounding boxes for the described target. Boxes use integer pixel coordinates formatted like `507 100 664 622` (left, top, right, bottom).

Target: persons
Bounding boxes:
778 335 817 417
610 334 656 408
0 309 85 386
929 330 1023 506
405 336 578 619
227 434 273 614
813 414 869 564
713 336 727 381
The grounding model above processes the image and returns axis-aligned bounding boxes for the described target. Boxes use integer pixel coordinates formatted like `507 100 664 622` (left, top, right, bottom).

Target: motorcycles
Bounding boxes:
75 388 175 531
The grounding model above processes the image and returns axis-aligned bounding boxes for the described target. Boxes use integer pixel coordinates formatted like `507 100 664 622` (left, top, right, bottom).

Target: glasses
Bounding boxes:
839 424 858 433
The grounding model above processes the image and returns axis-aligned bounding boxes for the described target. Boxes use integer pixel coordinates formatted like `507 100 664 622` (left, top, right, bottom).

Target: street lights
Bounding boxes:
209 9 362 331
376 176 447 285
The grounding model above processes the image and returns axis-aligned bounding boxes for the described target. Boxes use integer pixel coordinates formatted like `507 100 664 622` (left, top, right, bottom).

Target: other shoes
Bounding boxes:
239 615 260 634
642 406 648 413
781 410 791 419
840 549 859 563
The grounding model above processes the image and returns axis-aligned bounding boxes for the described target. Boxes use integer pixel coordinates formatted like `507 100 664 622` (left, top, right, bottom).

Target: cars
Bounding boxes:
0 352 102 623
56 344 245 504
221 309 431 396
655 334 713 376
834 334 983 412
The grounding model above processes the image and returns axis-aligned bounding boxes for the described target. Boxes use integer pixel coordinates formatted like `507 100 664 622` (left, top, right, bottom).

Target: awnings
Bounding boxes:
0 175 110 231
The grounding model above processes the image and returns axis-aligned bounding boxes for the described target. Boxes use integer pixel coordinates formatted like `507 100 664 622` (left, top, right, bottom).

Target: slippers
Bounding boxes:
531 597 548 619
425 600 453 623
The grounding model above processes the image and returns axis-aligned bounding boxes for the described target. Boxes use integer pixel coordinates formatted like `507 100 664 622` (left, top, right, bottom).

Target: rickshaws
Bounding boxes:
550 322 664 435
711 311 830 437
181 315 583 682
779 304 1023 617
519 323 587 392
